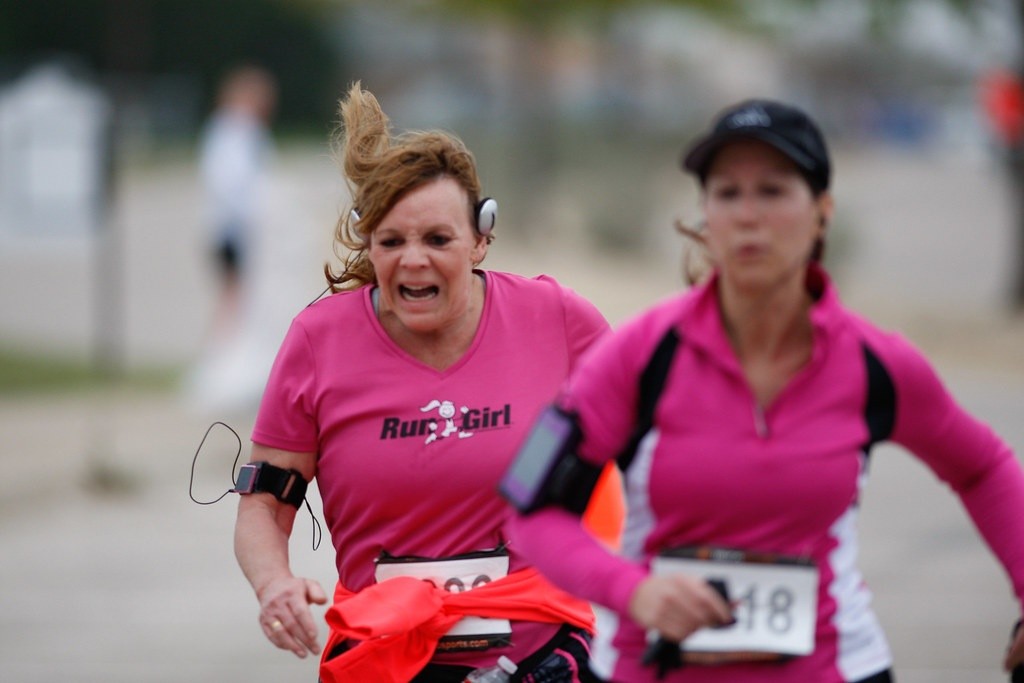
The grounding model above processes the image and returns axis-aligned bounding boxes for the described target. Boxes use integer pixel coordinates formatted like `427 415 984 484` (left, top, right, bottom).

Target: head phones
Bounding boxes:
351 196 498 241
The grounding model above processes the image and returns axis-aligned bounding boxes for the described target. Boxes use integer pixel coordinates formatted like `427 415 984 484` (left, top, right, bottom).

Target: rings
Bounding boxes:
270 621 281 628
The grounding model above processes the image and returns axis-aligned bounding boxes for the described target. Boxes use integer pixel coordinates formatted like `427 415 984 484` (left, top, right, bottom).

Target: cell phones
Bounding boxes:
497 407 581 512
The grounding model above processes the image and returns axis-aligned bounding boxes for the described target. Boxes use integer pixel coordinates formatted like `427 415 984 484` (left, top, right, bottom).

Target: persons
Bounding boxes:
233 80 659 683
181 74 278 401
503 98 1023 683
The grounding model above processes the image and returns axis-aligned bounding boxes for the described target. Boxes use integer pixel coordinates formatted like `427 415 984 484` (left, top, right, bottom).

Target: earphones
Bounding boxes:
818 212 827 227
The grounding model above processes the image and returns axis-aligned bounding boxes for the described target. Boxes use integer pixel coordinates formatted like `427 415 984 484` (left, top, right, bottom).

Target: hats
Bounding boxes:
683 99 831 196
